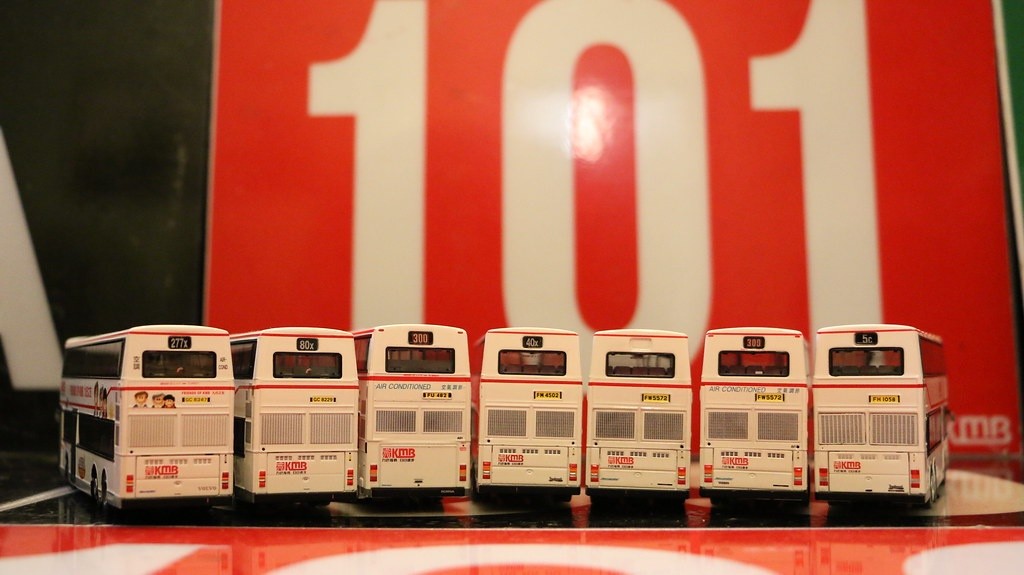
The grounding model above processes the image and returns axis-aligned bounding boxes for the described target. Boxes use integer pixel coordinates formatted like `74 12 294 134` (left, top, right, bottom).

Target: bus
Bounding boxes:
60 323 948 511
